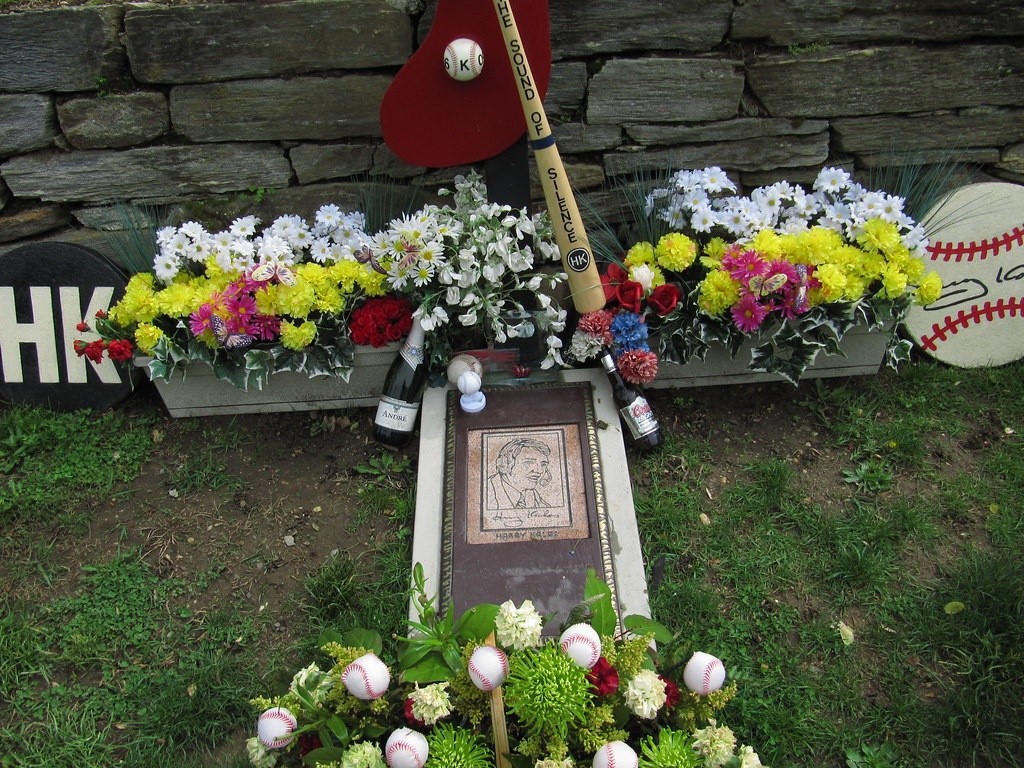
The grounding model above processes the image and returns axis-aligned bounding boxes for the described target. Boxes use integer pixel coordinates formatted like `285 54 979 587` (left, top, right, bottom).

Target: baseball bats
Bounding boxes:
491 0 609 316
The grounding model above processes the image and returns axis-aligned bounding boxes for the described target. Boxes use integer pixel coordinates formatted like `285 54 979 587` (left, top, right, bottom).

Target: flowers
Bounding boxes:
72 169 945 384
244 560 770 768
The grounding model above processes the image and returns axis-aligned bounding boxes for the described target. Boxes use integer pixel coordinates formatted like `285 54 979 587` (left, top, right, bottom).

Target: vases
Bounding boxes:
155 320 893 423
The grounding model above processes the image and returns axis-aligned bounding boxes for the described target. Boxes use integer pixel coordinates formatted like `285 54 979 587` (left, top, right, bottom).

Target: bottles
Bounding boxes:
599 345 663 449
372 317 428 446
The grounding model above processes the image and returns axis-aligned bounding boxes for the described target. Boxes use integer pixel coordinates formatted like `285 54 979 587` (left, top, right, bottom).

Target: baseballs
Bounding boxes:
256 623 727 768
447 354 484 388
457 370 483 395
443 36 486 83
